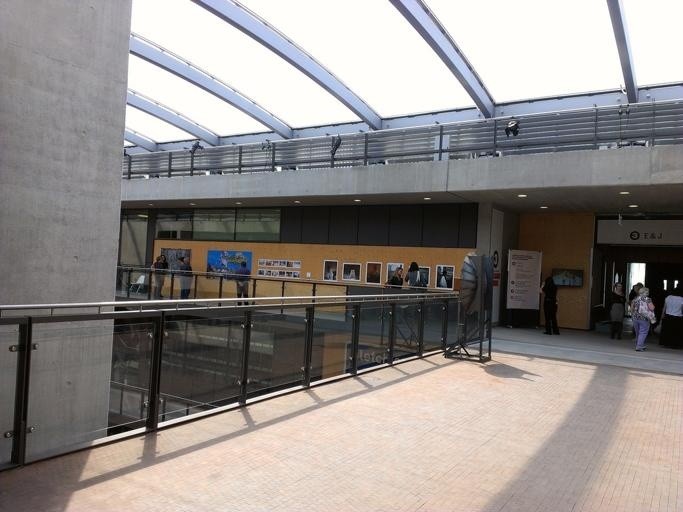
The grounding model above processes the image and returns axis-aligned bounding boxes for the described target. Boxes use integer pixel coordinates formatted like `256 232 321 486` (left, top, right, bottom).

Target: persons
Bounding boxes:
629 283 683 351
389 267 403 288
152 255 192 299
404 261 423 288
610 283 626 340
234 261 249 305
539 276 560 335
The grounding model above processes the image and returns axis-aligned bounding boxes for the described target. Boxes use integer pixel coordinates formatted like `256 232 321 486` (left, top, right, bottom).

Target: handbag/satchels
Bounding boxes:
410 280 427 290
638 300 655 320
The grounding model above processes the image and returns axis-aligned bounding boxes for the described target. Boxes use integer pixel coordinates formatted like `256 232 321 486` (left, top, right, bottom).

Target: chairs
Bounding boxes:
130 274 145 297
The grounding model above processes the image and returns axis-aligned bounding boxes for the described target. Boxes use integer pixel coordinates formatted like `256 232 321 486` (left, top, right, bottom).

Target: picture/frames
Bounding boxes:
435 264 455 291
342 262 362 281
407 265 431 287
365 261 382 285
257 258 302 279
386 263 406 286
322 260 338 282
